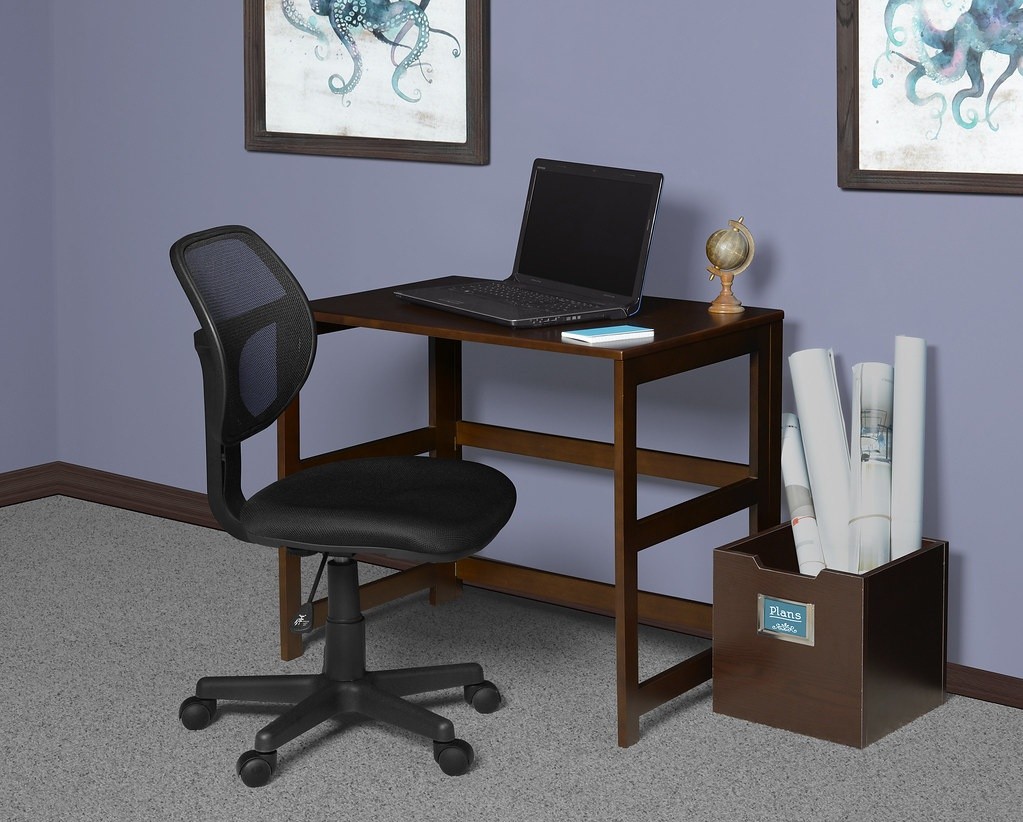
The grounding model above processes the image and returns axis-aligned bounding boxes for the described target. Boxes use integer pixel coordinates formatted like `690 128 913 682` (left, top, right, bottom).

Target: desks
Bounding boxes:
266 274 785 748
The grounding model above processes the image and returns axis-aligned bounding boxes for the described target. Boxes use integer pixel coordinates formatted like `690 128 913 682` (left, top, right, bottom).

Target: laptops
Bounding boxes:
393 158 664 327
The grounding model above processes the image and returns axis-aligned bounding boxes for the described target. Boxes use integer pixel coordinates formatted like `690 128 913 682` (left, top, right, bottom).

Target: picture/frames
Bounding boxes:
241 0 492 167
836 0 1023 196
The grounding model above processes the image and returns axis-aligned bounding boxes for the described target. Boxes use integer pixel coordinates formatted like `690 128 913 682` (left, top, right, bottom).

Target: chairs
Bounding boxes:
167 221 519 788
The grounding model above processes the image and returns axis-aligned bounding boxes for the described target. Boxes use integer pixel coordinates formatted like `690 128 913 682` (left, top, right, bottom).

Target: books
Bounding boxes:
561 324 654 345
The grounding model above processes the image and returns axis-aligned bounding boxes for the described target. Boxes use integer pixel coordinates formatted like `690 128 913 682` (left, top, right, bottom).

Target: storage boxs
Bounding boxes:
712 520 952 750
